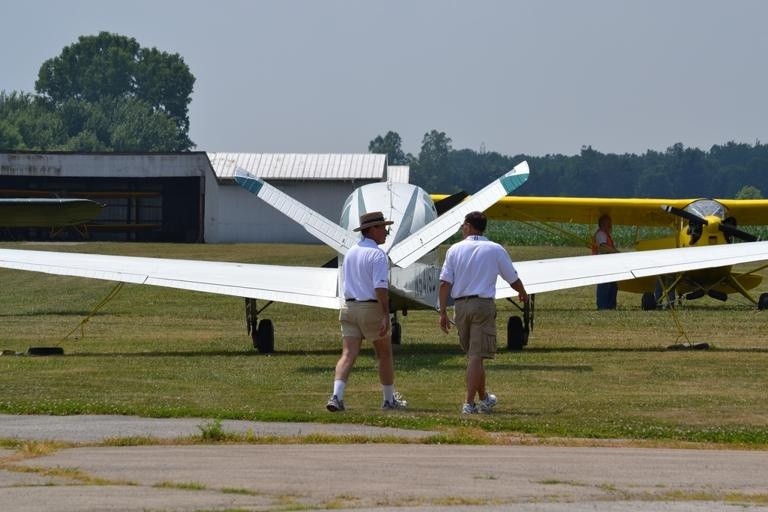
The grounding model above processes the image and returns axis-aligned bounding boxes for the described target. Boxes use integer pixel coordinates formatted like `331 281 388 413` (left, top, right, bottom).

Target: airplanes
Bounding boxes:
425 190 767 315
0 154 768 355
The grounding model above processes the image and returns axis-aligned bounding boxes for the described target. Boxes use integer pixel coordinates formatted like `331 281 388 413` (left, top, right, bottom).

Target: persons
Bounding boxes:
438 210 528 415
592 214 620 311
322 211 408 413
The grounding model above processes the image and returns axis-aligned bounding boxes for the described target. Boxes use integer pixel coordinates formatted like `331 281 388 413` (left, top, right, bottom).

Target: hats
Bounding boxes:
352 211 394 231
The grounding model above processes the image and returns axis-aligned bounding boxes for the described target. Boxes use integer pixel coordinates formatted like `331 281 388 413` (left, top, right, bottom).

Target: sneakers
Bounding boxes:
382 395 407 410
326 396 345 412
478 394 497 414
462 402 479 414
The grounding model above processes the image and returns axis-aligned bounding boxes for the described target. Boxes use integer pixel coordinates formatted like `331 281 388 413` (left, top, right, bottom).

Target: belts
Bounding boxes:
454 295 494 301
345 298 378 303
